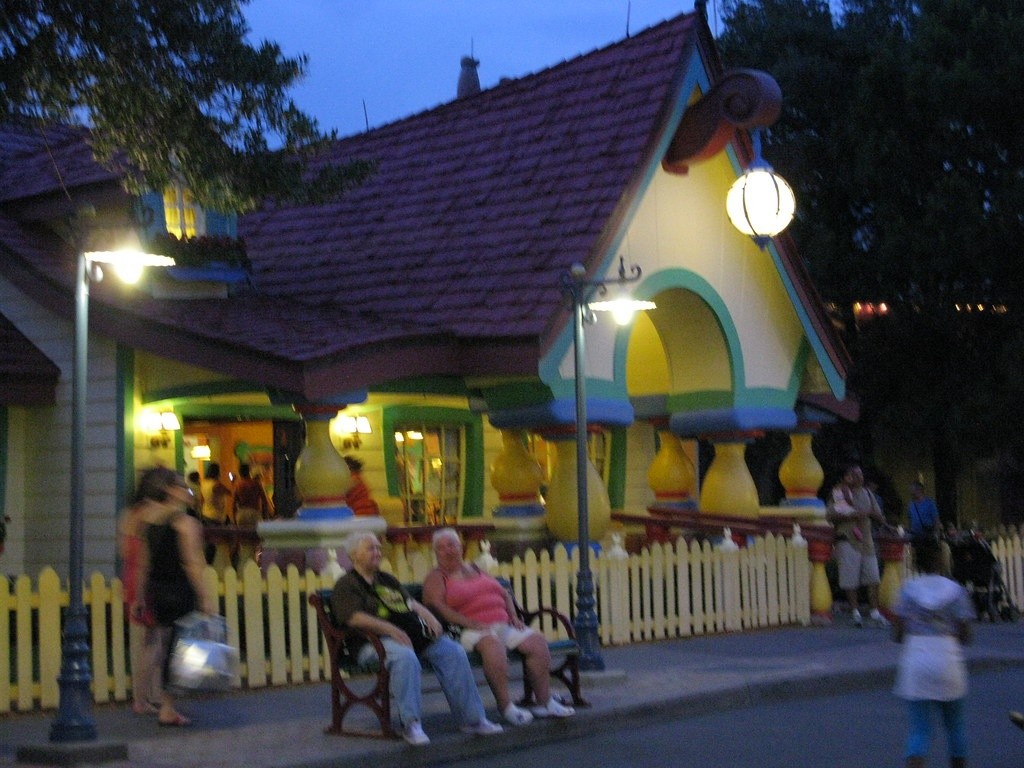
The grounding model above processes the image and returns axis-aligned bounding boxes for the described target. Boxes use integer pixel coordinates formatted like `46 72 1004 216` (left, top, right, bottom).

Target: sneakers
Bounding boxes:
502 702 534 725
402 719 429 745
533 699 576 717
463 719 503 735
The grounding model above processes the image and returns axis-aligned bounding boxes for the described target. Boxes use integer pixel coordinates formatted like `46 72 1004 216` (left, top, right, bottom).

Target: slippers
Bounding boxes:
159 714 192 727
133 703 160 715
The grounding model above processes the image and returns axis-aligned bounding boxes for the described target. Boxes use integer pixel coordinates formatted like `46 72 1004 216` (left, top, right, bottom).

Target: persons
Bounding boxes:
421 527 575 727
330 530 503 745
343 456 380 516
892 525 979 768
907 480 943 534
120 461 269 727
826 464 865 544
826 460 983 629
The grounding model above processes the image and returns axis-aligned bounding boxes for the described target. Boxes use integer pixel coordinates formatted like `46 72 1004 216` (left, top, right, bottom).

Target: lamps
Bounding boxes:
334 413 371 448
136 408 180 448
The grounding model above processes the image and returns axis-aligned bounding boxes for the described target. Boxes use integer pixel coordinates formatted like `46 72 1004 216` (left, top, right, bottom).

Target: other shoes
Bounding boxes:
1010 711 1024 728
950 757 964 768
867 616 891 628
908 758 925 768
853 616 863 629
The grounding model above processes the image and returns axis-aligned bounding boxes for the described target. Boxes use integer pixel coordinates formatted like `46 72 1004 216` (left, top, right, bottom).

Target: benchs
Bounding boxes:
308 576 590 742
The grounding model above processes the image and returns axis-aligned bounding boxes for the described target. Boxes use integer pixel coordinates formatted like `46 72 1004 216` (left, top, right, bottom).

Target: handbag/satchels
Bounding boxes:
389 610 432 655
171 639 237 692
919 527 939 561
